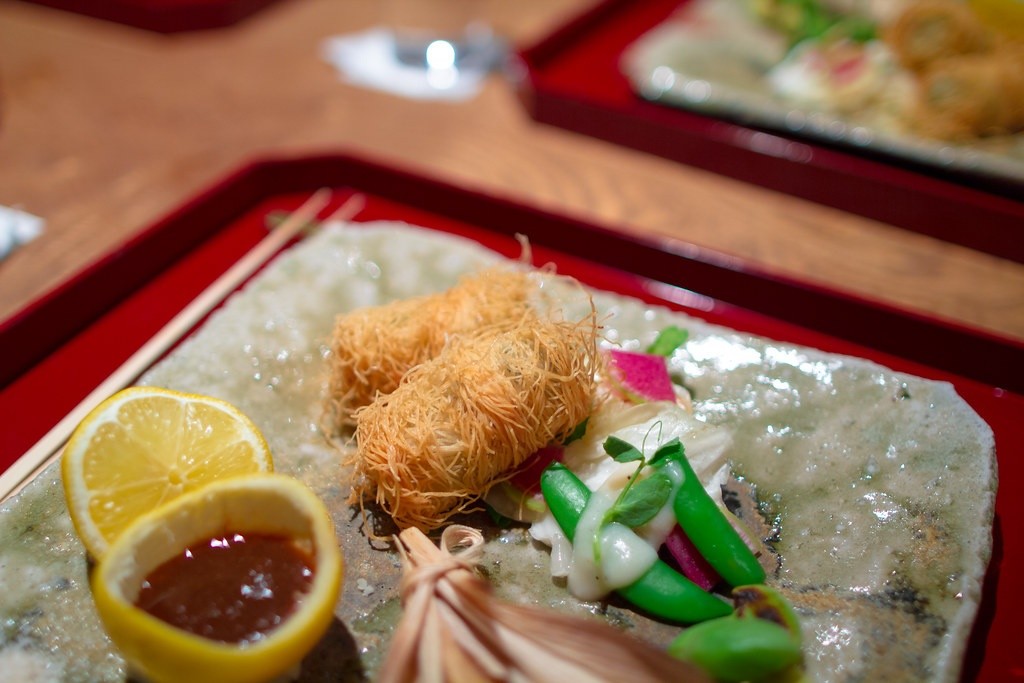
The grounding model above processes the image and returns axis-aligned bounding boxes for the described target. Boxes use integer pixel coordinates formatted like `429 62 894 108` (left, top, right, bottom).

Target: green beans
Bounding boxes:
664 613 801 683
657 453 765 587
538 461 733 623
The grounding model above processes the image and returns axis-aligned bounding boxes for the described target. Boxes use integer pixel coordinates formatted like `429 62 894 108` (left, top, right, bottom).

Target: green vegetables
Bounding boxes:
591 418 685 564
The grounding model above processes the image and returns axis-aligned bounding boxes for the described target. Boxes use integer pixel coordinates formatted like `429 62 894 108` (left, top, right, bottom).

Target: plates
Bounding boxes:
1 221 998 683
626 0 1023 180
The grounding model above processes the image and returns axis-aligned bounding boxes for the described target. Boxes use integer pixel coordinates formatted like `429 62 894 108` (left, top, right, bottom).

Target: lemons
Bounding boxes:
60 385 272 568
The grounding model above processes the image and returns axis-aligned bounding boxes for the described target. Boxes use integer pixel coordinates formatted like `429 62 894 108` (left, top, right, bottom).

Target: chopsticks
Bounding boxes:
0 186 364 505
